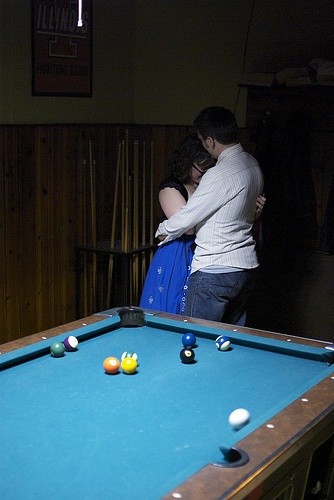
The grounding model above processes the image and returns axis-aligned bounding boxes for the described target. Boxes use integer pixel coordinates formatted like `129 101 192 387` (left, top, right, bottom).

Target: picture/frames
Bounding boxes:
30 0 95 98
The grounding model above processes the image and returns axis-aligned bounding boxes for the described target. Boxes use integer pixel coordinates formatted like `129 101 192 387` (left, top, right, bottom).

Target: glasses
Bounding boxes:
188 162 208 176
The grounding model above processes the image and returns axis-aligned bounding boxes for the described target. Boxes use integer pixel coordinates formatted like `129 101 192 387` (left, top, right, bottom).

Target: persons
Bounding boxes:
141 130 266 330
152 106 266 322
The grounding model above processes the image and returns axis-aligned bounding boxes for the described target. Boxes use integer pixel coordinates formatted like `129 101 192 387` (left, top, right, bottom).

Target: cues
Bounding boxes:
82 129 153 318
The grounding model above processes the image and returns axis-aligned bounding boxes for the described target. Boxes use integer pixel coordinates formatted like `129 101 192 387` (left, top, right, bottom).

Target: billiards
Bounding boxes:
228 408 250 430
50 342 65 357
180 348 195 362
182 333 196 347
103 357 120 374
63 336 78 351
121 357 137 374
215 335 231 350
